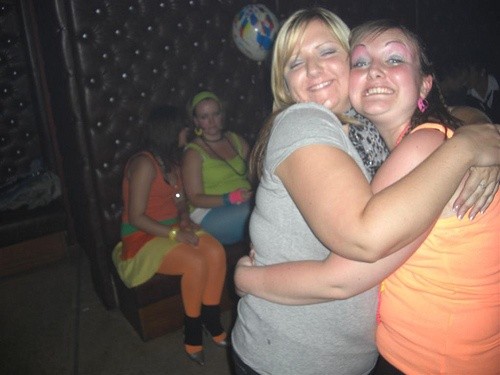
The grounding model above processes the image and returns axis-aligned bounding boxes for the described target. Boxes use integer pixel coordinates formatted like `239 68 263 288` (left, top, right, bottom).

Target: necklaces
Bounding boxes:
199 131 248 176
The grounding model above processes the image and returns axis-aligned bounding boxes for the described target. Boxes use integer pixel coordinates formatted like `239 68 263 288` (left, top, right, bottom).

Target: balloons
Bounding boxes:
231 3 281 66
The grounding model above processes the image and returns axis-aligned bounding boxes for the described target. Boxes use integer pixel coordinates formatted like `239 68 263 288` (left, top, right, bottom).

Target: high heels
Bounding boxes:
202 322 231 347
184 347 205 366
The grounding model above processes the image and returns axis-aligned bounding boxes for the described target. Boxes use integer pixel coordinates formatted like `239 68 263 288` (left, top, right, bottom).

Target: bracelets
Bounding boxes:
169 226 179 241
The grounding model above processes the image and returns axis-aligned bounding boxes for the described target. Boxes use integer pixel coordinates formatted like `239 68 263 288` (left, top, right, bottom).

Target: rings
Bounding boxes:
479 183 486 188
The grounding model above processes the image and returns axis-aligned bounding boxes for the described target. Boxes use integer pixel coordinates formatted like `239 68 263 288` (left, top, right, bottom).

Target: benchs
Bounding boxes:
0 205 70 278
108 237 250 343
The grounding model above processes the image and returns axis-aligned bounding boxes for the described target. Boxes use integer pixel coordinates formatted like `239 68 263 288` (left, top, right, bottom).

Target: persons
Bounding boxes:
228 6 500 375
426 58 499 121
233 17 500 375
181 90 260 246
111 105 230 366
